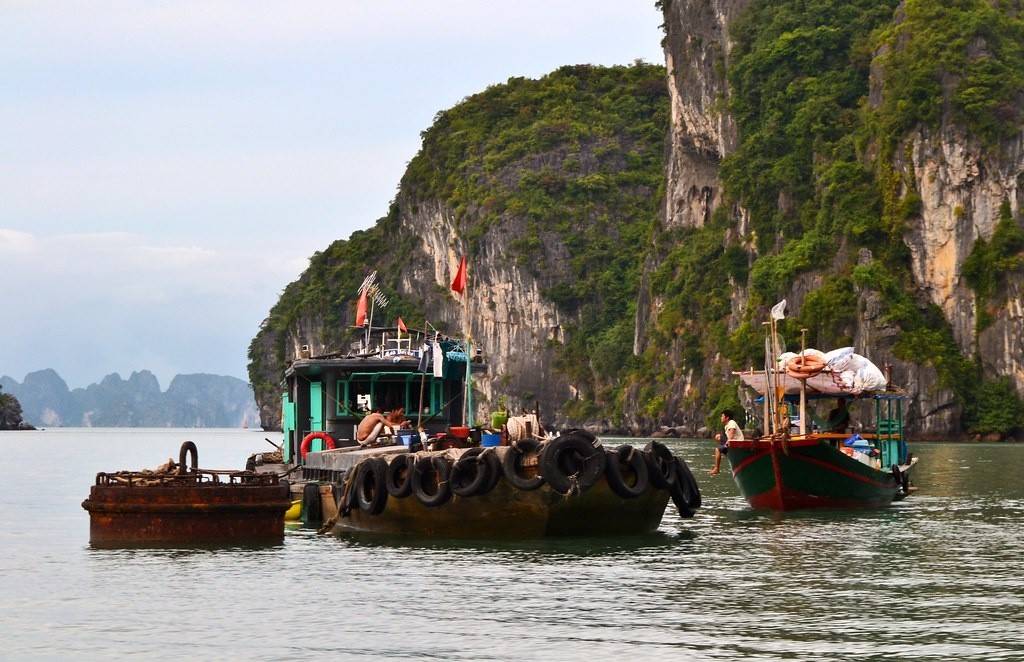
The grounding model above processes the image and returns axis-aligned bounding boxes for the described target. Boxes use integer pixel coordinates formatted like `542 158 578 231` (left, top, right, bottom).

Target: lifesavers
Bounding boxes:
605 441 701 518
300 431 335 460
302 430 605 524
892 464 909 492
787 355 827 378
437 434 462 450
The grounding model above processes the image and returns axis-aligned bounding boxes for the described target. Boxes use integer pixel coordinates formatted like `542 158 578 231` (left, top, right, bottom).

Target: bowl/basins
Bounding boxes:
401 434 430 446
449 427 469 438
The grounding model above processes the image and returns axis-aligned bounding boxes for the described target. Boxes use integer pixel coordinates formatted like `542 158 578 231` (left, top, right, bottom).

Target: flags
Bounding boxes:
356 291 368 326
451 258 465 297
398 318 407 336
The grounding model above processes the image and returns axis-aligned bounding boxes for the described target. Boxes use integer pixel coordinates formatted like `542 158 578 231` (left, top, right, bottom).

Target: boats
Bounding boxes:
246 272 702 541
722 301 921 513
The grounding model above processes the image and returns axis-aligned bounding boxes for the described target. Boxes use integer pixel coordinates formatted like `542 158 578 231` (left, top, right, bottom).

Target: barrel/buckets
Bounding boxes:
483 434 500 446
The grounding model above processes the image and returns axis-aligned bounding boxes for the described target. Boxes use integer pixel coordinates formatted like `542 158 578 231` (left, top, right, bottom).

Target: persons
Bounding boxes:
387 404 410 429
356 407 395 447
827 399 850 447
710 409 744 474
